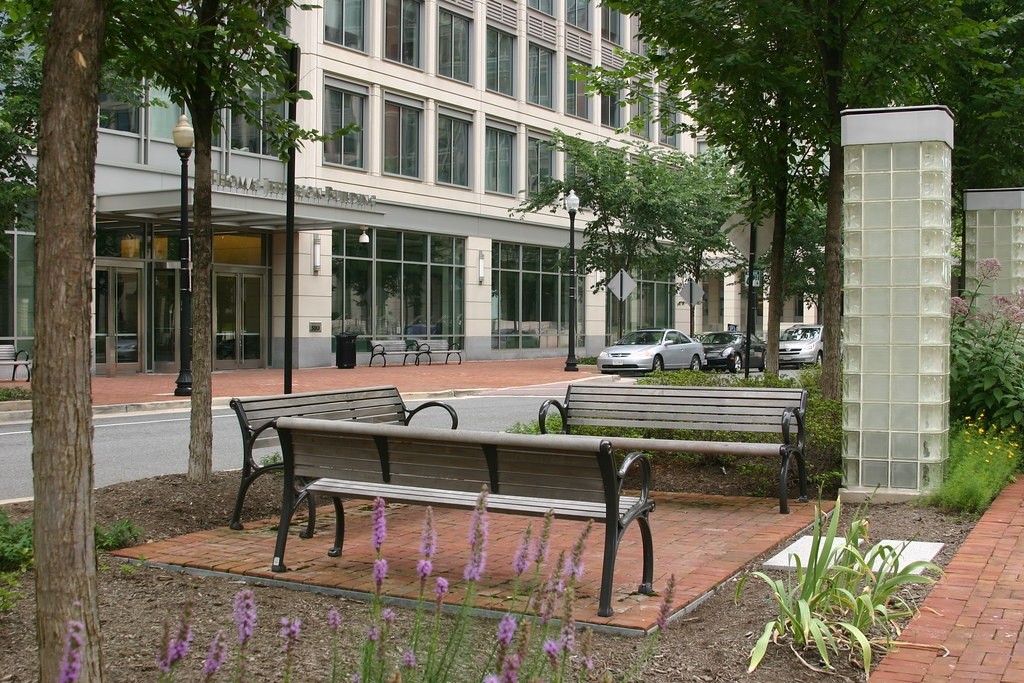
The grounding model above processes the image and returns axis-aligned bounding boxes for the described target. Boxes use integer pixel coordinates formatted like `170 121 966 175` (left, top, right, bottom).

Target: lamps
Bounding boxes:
359 225 369 243
313 234 322 272
478 252 485 284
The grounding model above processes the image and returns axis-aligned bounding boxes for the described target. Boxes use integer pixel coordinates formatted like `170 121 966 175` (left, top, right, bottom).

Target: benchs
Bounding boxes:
272 418 657 618
229 386 458 538
539 384 809 513
0 344 31 382
368 339 427 367
412 340 462 365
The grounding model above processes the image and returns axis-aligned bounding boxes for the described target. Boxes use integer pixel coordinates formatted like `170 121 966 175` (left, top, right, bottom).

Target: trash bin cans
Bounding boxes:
334 331 359 369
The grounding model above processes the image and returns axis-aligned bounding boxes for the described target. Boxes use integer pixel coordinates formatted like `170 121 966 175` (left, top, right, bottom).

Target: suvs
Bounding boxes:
775 324 844 371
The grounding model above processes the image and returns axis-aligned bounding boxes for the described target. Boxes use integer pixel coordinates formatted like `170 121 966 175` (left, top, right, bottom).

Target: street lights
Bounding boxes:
564 188 580 374
173 113 199 399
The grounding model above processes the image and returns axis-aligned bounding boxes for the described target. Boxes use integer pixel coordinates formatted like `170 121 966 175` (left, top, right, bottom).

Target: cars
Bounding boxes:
700 330 768 375
596 329 706 375
